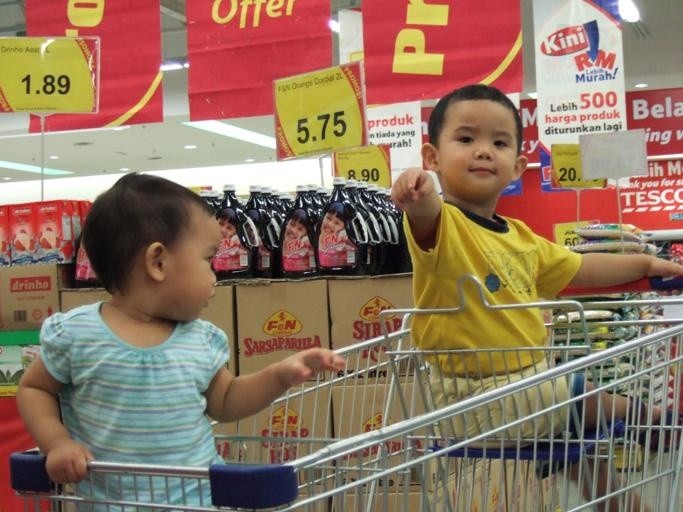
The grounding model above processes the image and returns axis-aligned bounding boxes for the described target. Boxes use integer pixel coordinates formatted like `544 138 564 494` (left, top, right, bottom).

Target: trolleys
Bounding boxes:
8 296 681 512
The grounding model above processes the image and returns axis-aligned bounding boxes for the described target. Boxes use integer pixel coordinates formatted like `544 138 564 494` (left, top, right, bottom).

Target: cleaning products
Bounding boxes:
68 229 102 288
188 178 425 276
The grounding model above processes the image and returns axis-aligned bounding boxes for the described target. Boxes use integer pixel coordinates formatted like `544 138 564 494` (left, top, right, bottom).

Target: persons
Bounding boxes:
14 172 344 512
284 210 307 240
216 209 235 240
321 203 344 235
392 84 682 512
